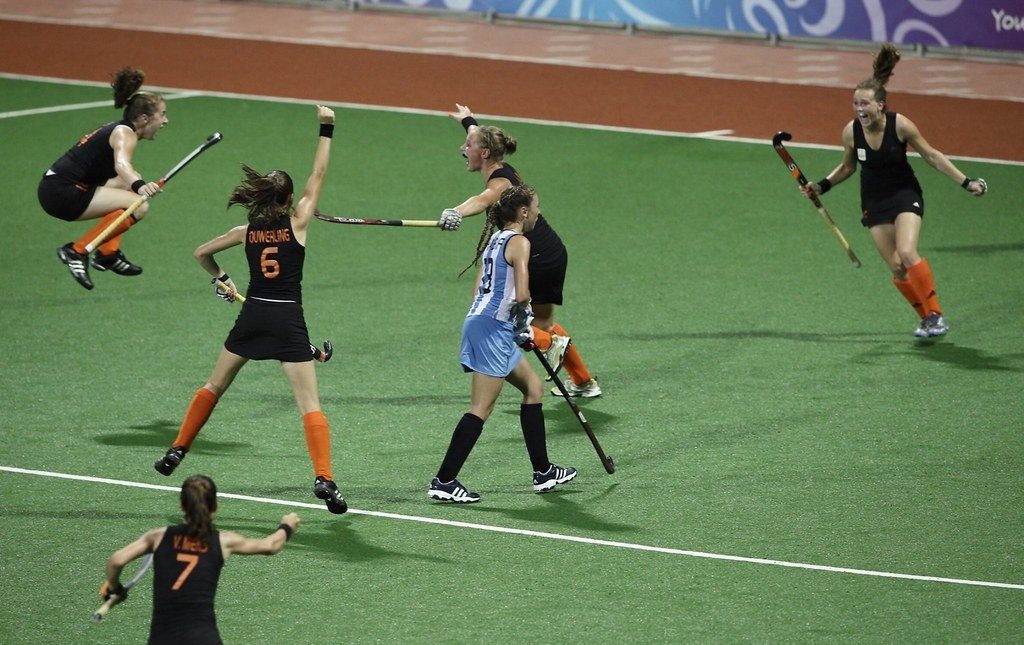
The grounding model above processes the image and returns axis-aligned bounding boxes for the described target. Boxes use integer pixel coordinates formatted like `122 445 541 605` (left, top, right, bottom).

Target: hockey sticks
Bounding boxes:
93 554 153 622
210 276 334 362
522 328 615 474
313 208 441 228
86 132 223 254
771 130 862 269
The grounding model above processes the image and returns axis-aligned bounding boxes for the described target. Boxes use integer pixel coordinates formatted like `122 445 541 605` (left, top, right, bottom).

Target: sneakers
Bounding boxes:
544 335 571 381
533 462 577 494
313 475 347 514
550 379 602 398
91 248 142 276
927 312 949 336
57 242 93 290
154 446 188 476
915 317 933 338
428 477 481 502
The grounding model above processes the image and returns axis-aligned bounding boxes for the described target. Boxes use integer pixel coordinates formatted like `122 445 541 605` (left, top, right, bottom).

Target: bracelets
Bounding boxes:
131 179 146 194
218 273 230 282
962 178 971 189
277 523 292 542
319 124 335 138
817 178 832 195
461 116 478 135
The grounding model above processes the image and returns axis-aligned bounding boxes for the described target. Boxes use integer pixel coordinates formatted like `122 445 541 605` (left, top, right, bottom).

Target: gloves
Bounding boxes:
511 311 535 351
961 178 988 196
97 581 128 605
209 271 238 302
439 206 462 230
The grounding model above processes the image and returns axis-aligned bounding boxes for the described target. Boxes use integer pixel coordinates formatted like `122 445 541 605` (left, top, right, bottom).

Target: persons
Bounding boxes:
38 65 169 290
427 186 578 504
154 104 348 515
437 103 602 399
103 474 300 645
798 41 988 337
